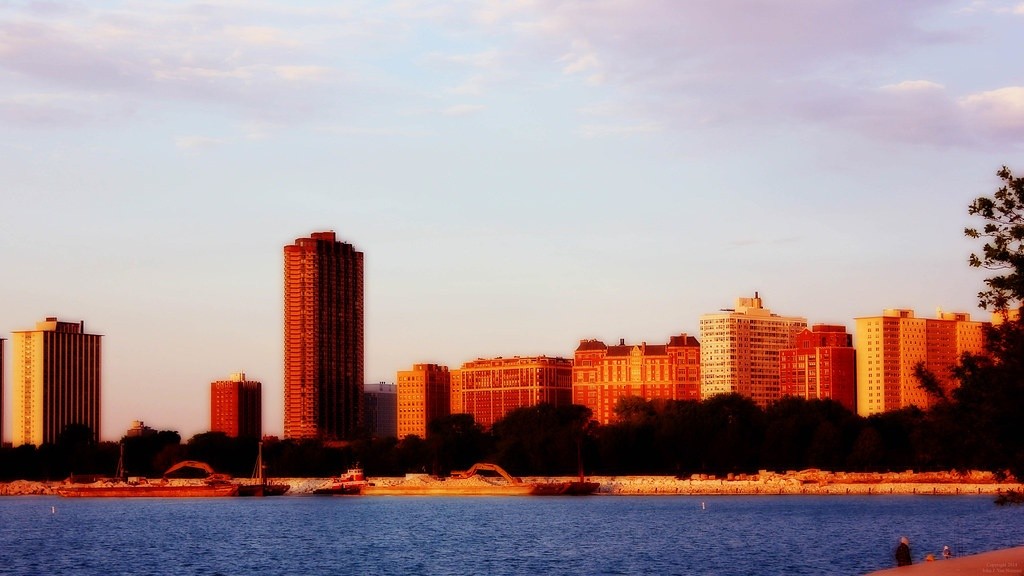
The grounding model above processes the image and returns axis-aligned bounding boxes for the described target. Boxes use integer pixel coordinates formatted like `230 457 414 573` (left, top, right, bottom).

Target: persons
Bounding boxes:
942 545 952 559
895 536 913 567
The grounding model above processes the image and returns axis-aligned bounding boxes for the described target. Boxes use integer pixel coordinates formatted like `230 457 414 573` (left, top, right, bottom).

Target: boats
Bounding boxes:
310 461 376 495
237 442 290 497
52 483 244 498
360 481 601 495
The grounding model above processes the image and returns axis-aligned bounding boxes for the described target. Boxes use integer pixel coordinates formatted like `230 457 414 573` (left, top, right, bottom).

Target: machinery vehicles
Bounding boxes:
450 463 527 486
114 444 127 483
764 468 824 483
158 460 233 485
